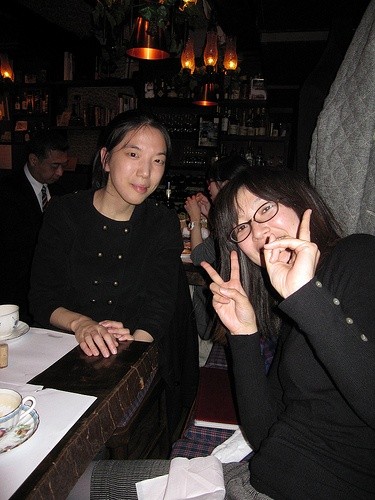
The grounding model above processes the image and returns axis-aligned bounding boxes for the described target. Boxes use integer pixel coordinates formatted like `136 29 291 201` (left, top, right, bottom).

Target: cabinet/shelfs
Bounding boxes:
0 70 299 201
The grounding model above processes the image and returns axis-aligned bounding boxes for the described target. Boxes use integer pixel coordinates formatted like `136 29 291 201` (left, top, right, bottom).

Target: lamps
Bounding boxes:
126 0 240 107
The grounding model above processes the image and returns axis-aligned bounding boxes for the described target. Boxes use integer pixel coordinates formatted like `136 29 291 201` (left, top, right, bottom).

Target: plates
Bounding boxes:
0 320 30 342
0 405 40 454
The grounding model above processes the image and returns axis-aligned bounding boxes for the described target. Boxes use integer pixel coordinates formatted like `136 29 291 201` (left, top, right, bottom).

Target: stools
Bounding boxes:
170 342 240 459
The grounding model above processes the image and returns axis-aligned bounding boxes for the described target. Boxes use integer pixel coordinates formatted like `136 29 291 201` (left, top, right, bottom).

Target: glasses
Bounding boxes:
207 178 225 184
230 198 283 246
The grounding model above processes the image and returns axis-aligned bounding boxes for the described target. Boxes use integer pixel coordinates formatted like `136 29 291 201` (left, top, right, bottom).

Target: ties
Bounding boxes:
40 186 48 213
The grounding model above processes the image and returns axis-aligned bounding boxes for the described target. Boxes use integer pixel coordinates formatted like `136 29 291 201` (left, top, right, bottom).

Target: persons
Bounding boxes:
65 161 375 500
184 156 250 341
29 107 186 358
0 129 79 324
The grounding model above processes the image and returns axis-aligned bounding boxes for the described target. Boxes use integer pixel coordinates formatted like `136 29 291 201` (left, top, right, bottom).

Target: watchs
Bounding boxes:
187 220 202 231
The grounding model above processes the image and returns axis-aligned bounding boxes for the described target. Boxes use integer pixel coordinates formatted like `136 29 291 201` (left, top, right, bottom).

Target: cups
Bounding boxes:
0 388 37 434
0 304 20 337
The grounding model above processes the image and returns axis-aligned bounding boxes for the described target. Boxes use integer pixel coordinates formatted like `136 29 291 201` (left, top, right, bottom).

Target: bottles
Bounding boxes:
64 66 289 222
14 90 49 142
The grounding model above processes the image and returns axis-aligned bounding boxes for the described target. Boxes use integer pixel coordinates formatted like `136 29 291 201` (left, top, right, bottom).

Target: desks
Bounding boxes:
0 318 162 500
